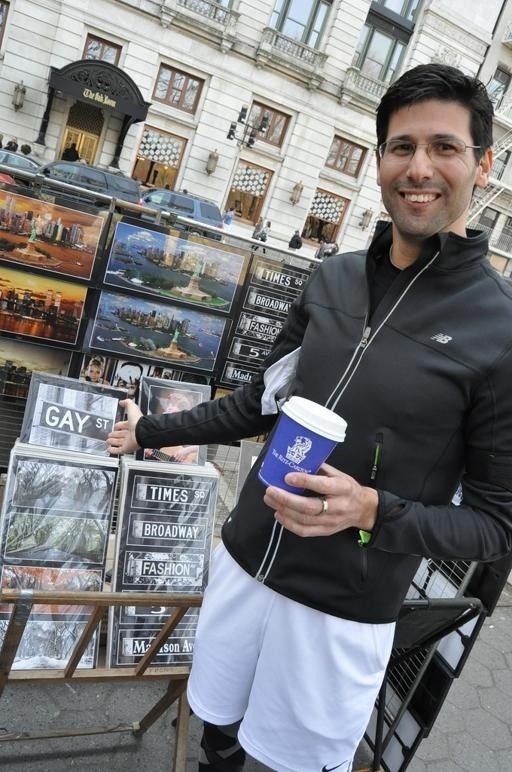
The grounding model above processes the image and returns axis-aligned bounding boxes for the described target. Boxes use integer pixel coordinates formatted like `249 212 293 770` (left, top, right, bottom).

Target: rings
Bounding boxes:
313 495 329 514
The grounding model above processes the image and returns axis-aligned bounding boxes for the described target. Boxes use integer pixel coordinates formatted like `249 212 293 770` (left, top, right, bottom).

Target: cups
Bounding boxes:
256 394 348 496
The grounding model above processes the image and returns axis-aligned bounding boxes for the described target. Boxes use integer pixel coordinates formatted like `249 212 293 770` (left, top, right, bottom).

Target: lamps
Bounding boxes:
12 80 27 111
290 180 305 207
206 149 219 176
358 207 374 232
227 105 270 151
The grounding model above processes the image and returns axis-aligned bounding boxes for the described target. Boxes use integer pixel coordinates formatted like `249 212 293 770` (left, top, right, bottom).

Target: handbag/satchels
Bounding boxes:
260 231 267 241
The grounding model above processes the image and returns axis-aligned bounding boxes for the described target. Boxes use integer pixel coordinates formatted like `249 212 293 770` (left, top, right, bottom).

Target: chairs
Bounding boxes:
352 596 484 772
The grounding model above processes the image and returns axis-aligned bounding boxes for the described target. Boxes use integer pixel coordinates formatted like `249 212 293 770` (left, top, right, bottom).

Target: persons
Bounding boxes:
0 134 31 155
61 143 80 162
88 359 110 385
106 64 512 772
223 207 339 260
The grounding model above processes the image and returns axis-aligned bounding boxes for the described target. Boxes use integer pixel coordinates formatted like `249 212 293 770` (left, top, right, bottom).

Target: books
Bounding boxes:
0 371 218 671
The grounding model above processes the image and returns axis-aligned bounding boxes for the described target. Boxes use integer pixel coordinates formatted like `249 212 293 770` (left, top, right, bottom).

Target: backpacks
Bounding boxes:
297 238 302 249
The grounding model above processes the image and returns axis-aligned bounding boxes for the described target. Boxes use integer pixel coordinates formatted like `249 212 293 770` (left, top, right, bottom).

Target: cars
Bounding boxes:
0 149 223 242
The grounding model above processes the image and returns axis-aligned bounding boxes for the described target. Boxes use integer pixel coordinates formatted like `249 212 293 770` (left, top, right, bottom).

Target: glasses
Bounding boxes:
373 138 484 163
